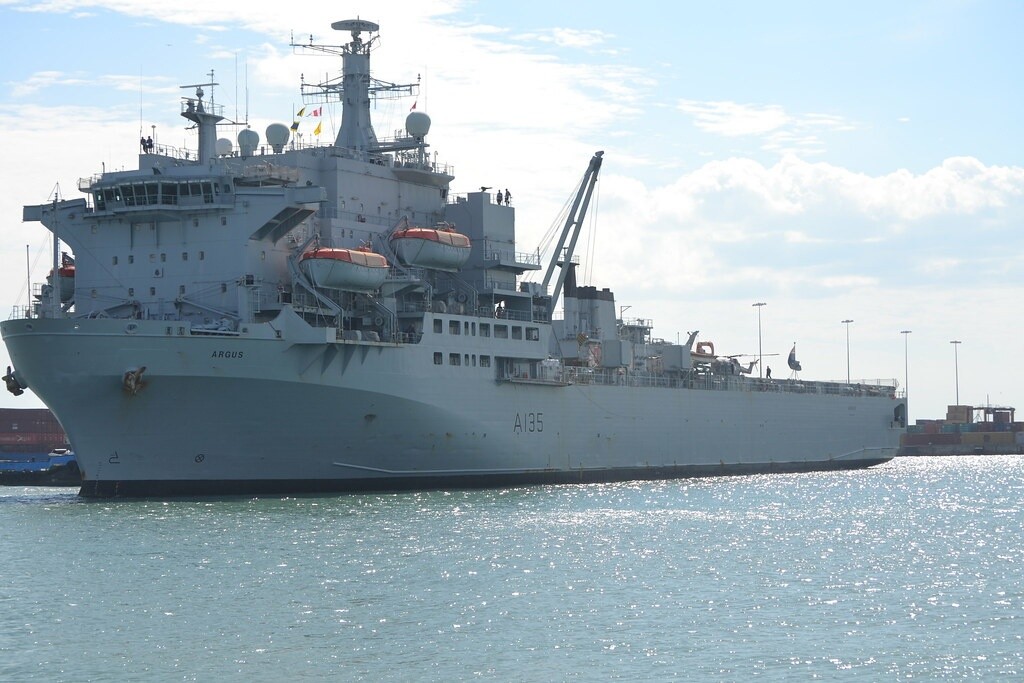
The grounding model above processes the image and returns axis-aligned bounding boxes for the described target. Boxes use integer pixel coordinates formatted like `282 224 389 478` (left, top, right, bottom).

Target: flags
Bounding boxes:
788 347 802 371
290 121 299 131
409 102 416 112
297 107 305 117
314 121 321 135
312 107 322 117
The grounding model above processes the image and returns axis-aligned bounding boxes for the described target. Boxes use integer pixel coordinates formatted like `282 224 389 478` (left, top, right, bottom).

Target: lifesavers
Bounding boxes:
892 394 896 399
375 316 383 326
458 293 466 303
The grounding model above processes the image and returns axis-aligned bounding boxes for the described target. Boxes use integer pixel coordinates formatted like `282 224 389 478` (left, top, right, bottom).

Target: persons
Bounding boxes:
496 304 502 316
141 137 148 154
497 189 502 205
766 366 772 378
147 136 153 152
505 189 511 204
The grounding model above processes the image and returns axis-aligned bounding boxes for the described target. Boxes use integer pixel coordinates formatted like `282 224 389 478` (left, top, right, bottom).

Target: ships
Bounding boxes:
0 16 908 497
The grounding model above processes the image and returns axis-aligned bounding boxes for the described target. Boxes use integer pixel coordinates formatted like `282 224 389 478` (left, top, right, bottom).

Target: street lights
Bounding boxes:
752 303 768 378
950 341 961 406
901 330 912 426
841 320 854 383
